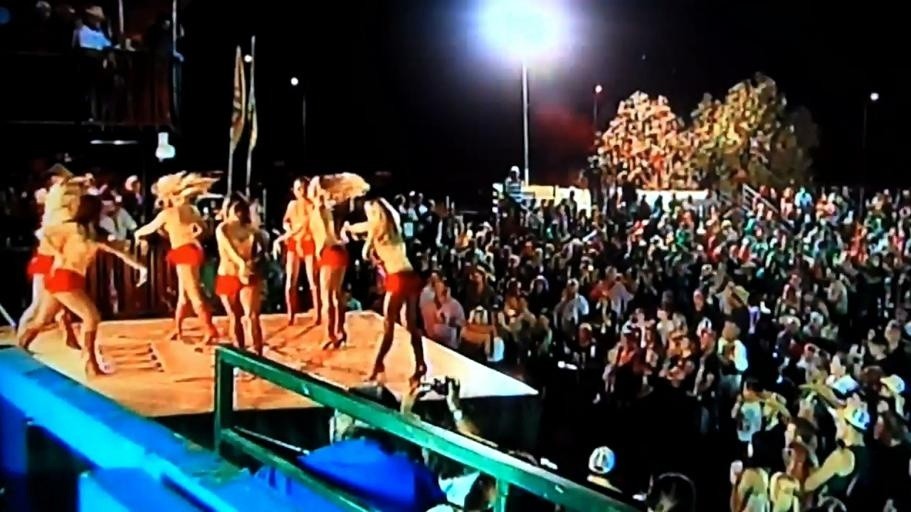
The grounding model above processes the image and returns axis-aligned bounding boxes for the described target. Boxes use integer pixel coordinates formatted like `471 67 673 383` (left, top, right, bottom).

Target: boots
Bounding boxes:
58 316 82 349
165 314 183 340
84 330 116 375
312 288 321 325
319 305 346 351
19 328 39 348
363 334 392 381
286 290 297 325
409 336 427 383
193 310 218 344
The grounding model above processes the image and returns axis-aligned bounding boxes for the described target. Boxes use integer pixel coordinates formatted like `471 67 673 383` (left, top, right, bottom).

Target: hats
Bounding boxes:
726 281 750 303
837 403 870 431
880 374 905 394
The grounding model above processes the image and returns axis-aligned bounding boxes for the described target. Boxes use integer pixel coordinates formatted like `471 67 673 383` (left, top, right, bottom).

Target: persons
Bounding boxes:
17 165 373 384
72 6 112 51
299 154 911 511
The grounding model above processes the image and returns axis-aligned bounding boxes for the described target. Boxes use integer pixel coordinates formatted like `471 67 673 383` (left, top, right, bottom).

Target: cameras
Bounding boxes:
416 376 461 398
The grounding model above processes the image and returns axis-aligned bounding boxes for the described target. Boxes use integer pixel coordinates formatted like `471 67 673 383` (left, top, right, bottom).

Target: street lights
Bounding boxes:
863 92 879 139
291 75 309 126
591 82 604 124
505 8 538 184
